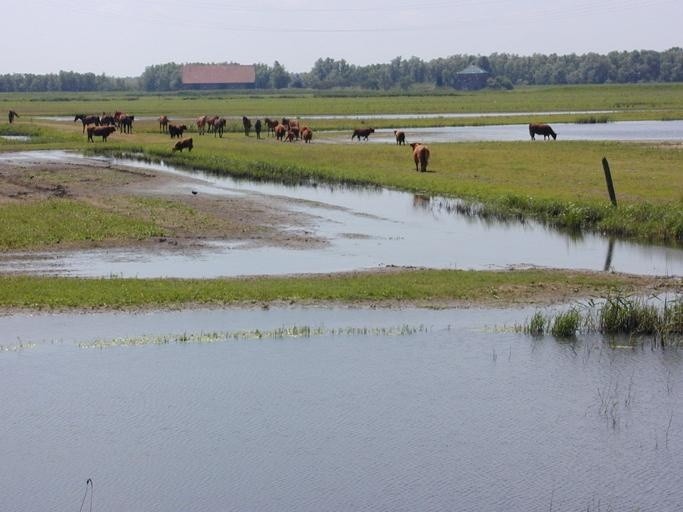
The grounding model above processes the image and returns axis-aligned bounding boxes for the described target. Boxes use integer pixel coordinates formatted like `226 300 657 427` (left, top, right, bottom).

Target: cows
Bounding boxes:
85 126 115 143
409 141 429 174
172 137 193 154
168 124 186 139
393 129 405 145
527 122 557 141
351 127 375 140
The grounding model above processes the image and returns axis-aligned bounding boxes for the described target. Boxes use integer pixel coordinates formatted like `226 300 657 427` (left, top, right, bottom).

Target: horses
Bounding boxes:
195 115 226 138
73 110 134 135
242 115 312 144
159 115 167 134
